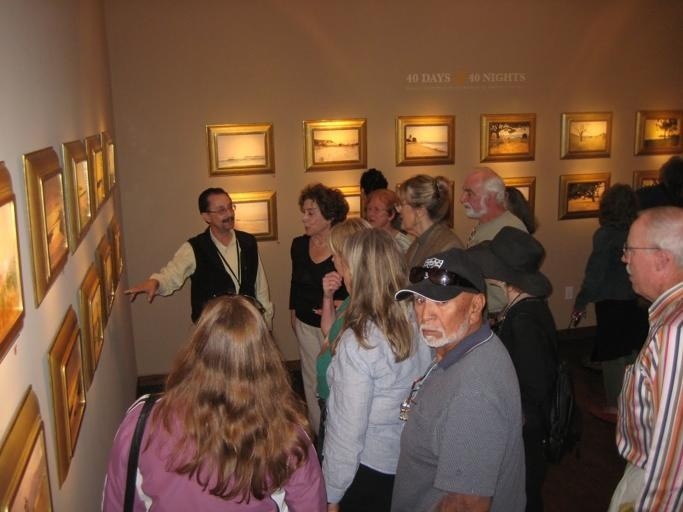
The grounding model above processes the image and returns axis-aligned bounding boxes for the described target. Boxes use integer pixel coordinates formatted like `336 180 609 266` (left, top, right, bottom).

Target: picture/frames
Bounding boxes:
395 114 456 167
394 177 457 230
503 176 538 208
333 184 365 225
228 191 280 243
560 112 614 160
479 113 538 163
558 172 612 220
205 122 278 179
633 108 683 159
632 170 665 194
304 118 370 172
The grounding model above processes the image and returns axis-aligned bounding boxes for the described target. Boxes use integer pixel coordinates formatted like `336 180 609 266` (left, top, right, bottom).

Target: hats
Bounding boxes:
464 225 553 298
393 248 486 302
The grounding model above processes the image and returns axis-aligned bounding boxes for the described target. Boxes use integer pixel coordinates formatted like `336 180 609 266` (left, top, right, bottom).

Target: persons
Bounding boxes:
124 187 274 336
101 295 328 511
289 167 683 511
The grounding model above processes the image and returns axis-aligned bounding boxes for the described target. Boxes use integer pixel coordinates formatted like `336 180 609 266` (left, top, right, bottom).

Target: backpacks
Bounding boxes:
506 308 575 465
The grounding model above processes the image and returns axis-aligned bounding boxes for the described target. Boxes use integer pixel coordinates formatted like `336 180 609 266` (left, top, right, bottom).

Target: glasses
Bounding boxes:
208 205 236 215
408 266 480 293
398 381 415 422
620 241 663 255
392 202 424 214
